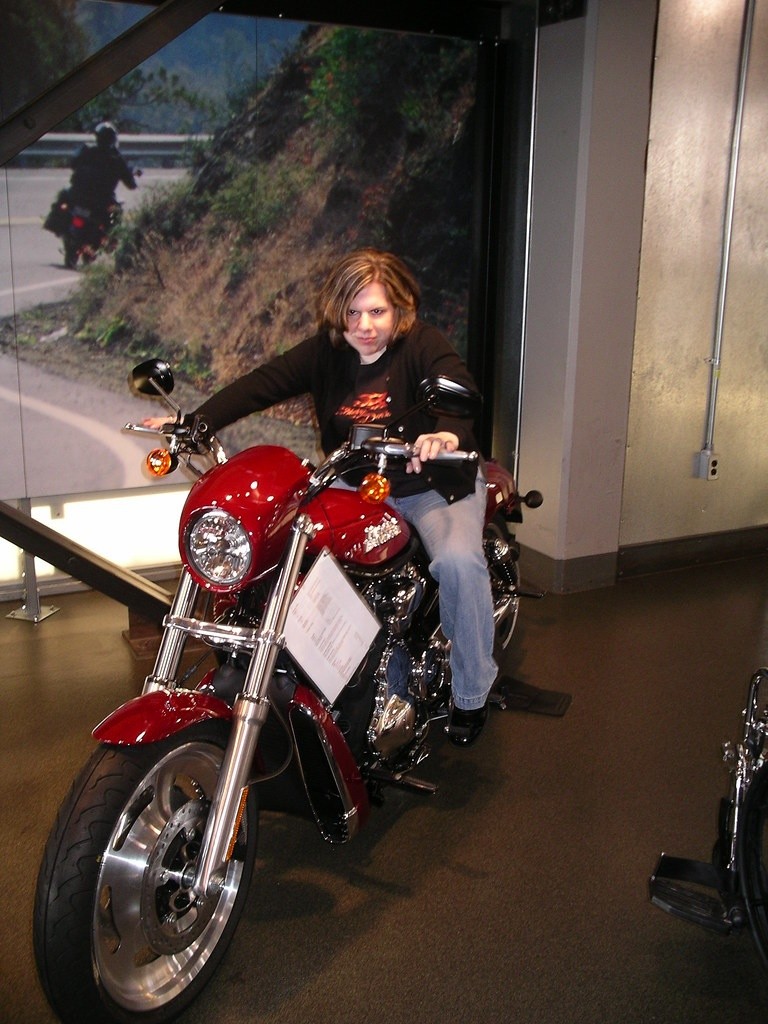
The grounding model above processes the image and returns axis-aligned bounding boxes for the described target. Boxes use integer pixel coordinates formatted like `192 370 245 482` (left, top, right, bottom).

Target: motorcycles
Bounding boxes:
44 157 142 267
30 360 544 1023
646 667 768 1019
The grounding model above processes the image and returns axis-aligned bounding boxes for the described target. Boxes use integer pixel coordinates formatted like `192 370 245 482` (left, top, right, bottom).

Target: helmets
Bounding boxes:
96 122 116 139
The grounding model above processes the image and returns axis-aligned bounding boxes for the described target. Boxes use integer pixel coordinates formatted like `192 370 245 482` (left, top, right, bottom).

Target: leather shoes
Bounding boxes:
450 704 488 748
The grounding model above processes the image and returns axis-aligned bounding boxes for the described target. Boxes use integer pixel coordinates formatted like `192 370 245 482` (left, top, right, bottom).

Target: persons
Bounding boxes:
57 119 136 256
139 245 499 747
